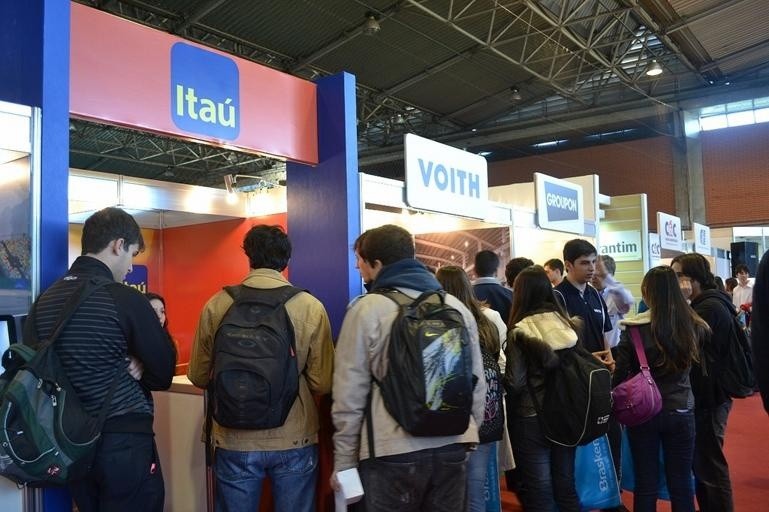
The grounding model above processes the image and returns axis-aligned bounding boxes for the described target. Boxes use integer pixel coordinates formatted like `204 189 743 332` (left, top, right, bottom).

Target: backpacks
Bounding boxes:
693 291 756 401
369 286 477 439
207 282 309 431
0 273 120 492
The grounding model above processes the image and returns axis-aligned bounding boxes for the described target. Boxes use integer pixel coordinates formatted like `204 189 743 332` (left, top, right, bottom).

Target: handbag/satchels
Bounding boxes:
573 434 624 510
537 345 615 450
619 425 696 502
479 349 505 442
610 366 662 428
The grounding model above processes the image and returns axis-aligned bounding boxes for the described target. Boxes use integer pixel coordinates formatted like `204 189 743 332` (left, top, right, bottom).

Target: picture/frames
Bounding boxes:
0 100 42 317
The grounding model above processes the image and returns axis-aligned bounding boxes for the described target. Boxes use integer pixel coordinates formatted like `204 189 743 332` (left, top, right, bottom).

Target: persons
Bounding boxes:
503 238 769 512
330 225 507 512
21 205 176 512
187 224 336 511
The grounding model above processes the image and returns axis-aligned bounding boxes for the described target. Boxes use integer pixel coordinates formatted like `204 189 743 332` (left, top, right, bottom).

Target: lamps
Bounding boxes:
164 165 175 178
509 84 522 102
364 8 381 33
227 151 238 163
646 55 662 76
